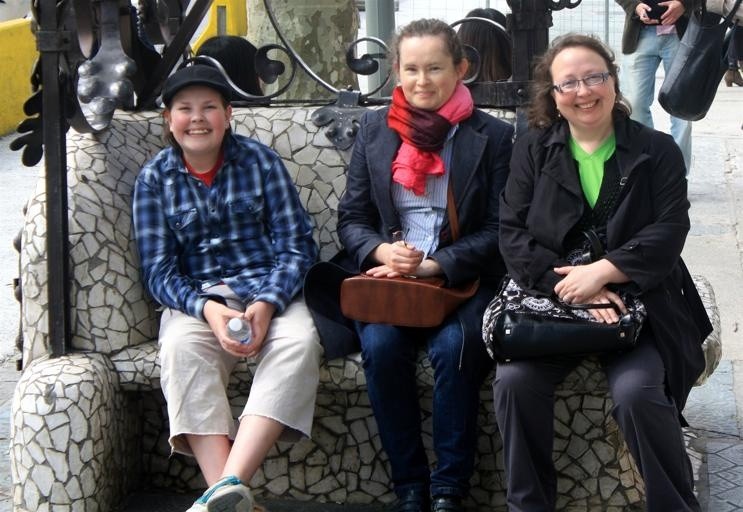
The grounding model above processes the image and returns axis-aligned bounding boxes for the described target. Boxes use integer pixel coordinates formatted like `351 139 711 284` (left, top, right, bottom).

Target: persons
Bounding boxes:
611 1 708 211
301 17 515 511
130 66 324 510
481 31 713 511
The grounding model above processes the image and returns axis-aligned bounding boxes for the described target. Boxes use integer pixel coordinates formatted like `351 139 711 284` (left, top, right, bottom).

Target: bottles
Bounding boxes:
224 317 252 344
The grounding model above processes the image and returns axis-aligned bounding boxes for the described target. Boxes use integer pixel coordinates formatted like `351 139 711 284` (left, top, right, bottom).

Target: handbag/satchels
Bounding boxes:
655 1 742 122
340 265 482 328
481 271 650 364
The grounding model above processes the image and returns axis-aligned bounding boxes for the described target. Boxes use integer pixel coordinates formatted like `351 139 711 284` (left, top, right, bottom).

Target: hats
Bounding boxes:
160 64 232 104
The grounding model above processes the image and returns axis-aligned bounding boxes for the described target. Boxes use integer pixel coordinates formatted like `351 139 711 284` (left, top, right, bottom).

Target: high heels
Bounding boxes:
724 70 743 87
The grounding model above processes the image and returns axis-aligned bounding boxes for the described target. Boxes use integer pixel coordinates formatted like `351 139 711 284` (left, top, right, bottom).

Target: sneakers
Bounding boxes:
392 490 462 512
187 472 257 511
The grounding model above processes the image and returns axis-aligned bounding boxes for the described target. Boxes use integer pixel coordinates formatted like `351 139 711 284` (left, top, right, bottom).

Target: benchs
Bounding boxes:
9 106 721 512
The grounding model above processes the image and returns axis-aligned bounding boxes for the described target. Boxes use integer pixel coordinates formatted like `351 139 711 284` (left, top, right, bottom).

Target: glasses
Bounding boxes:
548 71 614 95
